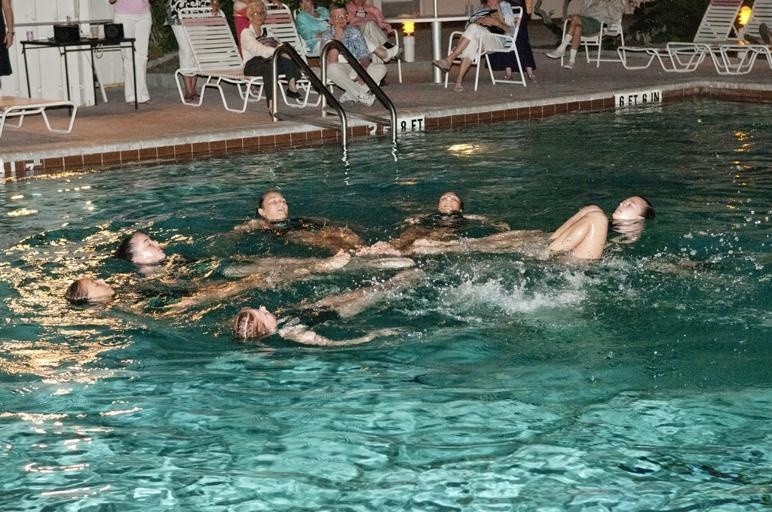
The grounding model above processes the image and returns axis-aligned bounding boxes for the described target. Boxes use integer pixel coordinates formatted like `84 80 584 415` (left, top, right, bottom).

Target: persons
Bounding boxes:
109 0 154 104
432 0 516 92
545 0 628 70
484 0 535 80
0 0 15 90
241 0 304 120
232 0 284 61
67 273 256 322
535 203 611 260
233 267 427 349
118 229 353 280
375 192 513 250
164 0 222 100
297 0 404 107
744 22 772 45
416 195 655 254
242 190 386 259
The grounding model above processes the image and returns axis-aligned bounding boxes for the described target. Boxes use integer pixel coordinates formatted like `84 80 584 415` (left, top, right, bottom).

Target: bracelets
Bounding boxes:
7 31 14 35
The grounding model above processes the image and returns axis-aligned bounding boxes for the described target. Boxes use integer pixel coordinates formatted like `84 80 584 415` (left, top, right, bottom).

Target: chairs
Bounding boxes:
166 1 401 113
617 1 744 70
716 1 772 75
444 5 528 92
561 2 627 68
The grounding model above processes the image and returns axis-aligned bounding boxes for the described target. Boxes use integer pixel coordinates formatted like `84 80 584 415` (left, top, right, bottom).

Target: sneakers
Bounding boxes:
545 51 564 59
285 88 303 99
563 61 575 71
382 45 398 64
340 94 376 107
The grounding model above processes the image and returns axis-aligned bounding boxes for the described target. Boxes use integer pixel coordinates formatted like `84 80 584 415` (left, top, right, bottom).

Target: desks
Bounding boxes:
667 41 738 73
382 12 474 85
19 34 140 116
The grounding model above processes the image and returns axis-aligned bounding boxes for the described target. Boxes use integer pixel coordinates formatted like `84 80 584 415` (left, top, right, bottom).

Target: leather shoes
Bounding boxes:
504 73 536 81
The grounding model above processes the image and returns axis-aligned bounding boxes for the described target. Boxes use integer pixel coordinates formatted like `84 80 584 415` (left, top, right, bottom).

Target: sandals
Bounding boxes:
454 82 465 94
431 58 452 73
183 92 202 103
